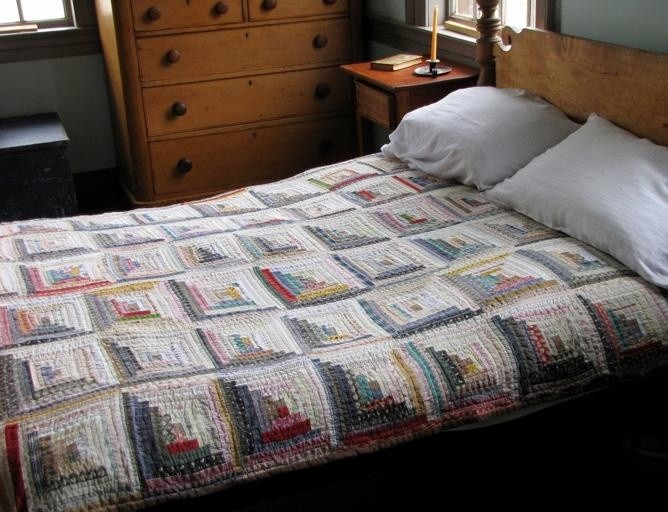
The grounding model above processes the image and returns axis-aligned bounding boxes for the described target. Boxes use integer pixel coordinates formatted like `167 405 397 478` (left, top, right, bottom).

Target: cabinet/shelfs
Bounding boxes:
92 0 365 206
0 112 75 222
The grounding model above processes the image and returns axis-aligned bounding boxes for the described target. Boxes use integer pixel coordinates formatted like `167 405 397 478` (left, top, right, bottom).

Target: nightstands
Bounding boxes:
339 51 482 157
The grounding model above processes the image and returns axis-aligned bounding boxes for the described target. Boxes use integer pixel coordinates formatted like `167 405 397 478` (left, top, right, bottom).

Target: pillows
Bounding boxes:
380 86 581 191
484 110 665 285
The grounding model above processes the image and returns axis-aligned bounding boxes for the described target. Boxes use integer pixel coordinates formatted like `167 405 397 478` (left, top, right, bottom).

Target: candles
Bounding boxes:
431 5 440 61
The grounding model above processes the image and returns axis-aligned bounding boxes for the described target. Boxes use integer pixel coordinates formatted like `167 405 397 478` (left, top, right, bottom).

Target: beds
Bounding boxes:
0 25 667 512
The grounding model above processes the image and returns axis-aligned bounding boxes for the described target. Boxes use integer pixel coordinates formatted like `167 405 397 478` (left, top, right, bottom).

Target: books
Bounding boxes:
370 53 423 72
